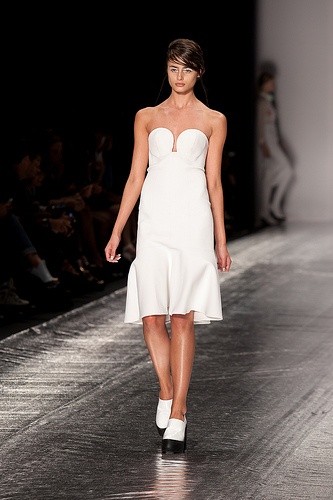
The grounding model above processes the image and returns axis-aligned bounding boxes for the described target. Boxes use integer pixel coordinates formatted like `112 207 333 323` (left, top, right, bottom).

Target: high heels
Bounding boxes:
155 391 174 436
161 416 187 452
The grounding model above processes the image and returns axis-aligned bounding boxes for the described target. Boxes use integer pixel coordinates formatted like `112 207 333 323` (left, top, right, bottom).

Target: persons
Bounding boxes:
0 123 138 315
104 38 232 455
254 72 293 226
